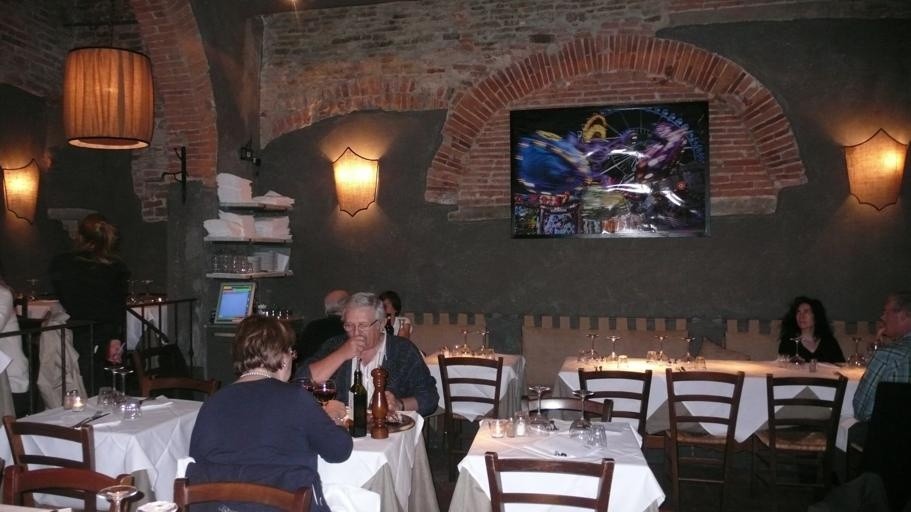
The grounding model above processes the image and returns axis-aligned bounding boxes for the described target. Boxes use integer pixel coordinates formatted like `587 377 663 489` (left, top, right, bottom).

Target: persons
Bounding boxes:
294 291 440 415
379 291 413 338
852 288 911 423
777 296 846 363
298 288 352 359
184 313 353 512
49 214 128 397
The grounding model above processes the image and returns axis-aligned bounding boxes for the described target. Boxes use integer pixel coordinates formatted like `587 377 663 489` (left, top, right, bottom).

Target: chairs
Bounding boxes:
752 373 850 512
2 411 100 511
2 463 134 511
436 352 504 480
174 478 313 512
522 394 615 424
664 368 744 512
806 470 886 512
579 365 653 462
136 374 220 403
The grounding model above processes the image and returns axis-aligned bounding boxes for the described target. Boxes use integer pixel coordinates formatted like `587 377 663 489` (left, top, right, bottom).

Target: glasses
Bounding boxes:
343 319 379 332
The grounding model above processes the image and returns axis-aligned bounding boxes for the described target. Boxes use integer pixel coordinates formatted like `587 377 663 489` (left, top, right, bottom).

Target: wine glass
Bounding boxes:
651 335 669 366
123 279 155 305
456 330 490 358
786 336 807 368
312 378 336 408
529 386 555 439
104 365 133 412
583 333 622 367
680 337 696 370
569 391 595 441
847 337 866 368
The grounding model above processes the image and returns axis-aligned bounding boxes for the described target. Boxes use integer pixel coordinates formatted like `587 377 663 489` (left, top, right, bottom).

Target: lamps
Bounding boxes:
2 158 42 228
840 127 910 213
62 0 159 153
326 145 381 221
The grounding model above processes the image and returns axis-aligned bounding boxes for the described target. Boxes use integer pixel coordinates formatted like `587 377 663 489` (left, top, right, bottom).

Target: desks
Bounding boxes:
239 404 443 512
552 352 869 447
421 353 527 426
17 390 207 509
449 409 668 512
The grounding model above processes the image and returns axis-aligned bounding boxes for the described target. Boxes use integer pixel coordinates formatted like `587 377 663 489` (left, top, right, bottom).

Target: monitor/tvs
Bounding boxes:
215 282 256 325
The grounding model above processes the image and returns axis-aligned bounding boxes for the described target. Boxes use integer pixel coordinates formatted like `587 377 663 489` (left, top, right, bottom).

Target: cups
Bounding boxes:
124 398 142 420
488 420 506 438
695 356 707 370
587 423 607 453
97 387 112 407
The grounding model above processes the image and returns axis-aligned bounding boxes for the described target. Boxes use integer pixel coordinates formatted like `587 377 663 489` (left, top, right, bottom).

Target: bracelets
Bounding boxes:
397 398 405 411
330 414 345 424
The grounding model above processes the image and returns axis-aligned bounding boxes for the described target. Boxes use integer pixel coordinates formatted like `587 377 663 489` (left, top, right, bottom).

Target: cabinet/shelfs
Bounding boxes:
205 200 307 363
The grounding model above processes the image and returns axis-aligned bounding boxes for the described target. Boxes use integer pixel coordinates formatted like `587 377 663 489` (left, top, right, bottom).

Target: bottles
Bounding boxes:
809 359 817 372
349 370 367 438
506 416 526 437
440 344 450 358
63 390 78 410
